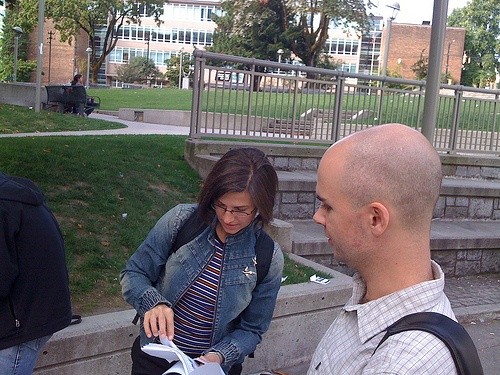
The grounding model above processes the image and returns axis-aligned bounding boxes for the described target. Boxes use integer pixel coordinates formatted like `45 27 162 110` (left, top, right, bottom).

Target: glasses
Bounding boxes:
211 201 256 217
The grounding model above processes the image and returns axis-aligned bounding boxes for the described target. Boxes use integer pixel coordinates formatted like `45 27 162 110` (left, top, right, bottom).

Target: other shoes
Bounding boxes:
82 111 88 118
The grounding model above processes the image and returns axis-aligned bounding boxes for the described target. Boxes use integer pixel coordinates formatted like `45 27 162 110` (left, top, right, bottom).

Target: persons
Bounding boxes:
274 122 483 375
0 172 72 375
72 74 98 117
118 149 284 375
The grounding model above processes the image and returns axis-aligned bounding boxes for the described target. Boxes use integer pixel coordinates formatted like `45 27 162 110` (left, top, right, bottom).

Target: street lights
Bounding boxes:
86 47 93 91
277 49 284 87
445 39 456 84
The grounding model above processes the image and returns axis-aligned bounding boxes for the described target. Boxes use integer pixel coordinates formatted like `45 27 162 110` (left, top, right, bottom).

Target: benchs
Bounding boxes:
44 84 101 118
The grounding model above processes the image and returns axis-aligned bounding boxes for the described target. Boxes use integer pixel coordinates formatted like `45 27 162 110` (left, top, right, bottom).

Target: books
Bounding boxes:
140 331 224 374
